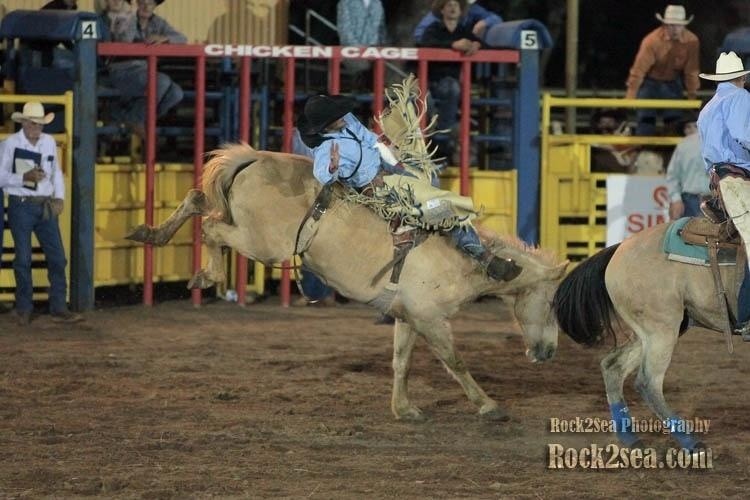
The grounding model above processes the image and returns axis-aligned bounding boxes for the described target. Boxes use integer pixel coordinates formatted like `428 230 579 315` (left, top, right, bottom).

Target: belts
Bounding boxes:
9 195 52 204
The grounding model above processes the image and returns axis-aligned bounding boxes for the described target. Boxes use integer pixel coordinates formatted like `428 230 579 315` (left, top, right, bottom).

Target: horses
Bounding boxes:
552 216 750 463
122 137 571 422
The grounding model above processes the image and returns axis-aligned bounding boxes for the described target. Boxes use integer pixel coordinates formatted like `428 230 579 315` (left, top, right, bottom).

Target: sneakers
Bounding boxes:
52 309 86 324
291 295 342 309
15 310 34 327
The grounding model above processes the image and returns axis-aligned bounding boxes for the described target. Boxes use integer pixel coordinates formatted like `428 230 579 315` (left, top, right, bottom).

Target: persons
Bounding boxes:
697 50 750 333
108 2 189 140
303 96 526 282
589 0 750 176
336 1 508 162
290 268 336 308
668 122 709 218
0 99 85 326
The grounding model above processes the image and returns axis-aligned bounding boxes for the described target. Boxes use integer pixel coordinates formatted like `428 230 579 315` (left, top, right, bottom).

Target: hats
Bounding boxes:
656 4 694 26
698 50 749 83
11 101 58 126
431 0 470 19
302 93 357 136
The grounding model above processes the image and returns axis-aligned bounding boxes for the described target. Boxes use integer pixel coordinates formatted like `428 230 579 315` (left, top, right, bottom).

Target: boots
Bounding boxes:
477 247 525 283
